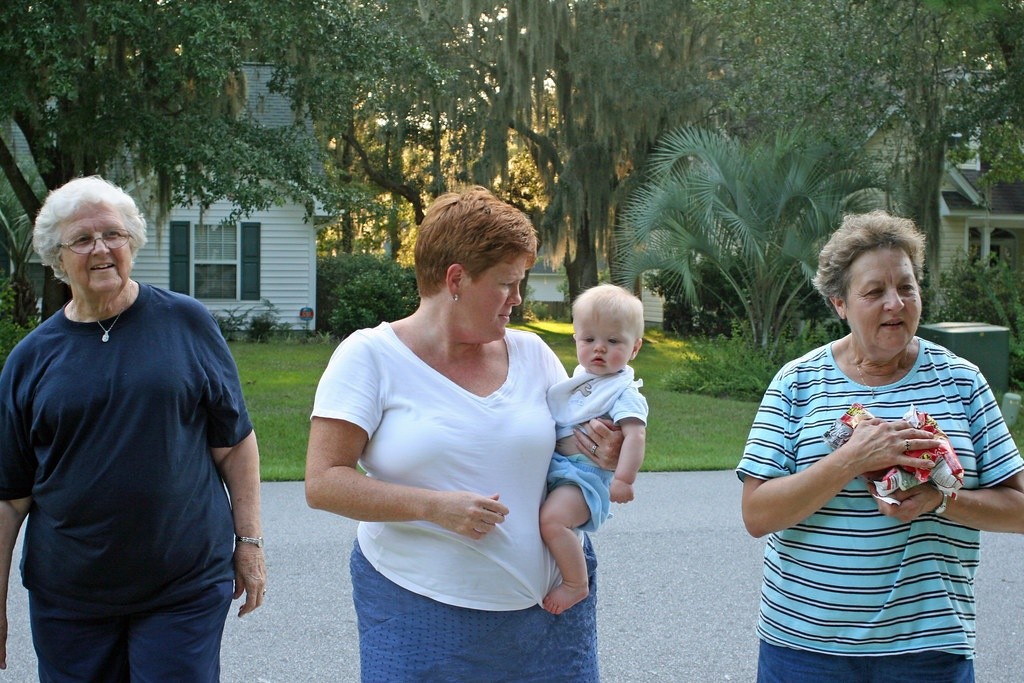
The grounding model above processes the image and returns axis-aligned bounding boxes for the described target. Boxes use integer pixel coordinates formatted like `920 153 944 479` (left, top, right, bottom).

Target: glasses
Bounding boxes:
57 229 129 254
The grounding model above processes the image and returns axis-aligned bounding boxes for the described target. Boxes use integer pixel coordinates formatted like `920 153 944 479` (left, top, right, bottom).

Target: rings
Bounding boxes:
905 439 910 451
591 445 598 454
263 589 266 595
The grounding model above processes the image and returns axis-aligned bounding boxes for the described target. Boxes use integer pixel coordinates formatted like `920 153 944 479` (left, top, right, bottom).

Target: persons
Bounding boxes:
540 284 648 615
0 174 267 683
304 186 624 683
736 210 1024 683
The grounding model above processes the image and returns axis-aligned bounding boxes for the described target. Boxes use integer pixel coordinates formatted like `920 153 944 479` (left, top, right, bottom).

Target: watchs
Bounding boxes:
235 535 264 549
936 492 947 517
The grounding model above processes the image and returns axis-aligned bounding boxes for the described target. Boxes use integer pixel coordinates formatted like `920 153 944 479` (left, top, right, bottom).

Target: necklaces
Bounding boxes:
851 334 909 399
97 280 132 342
